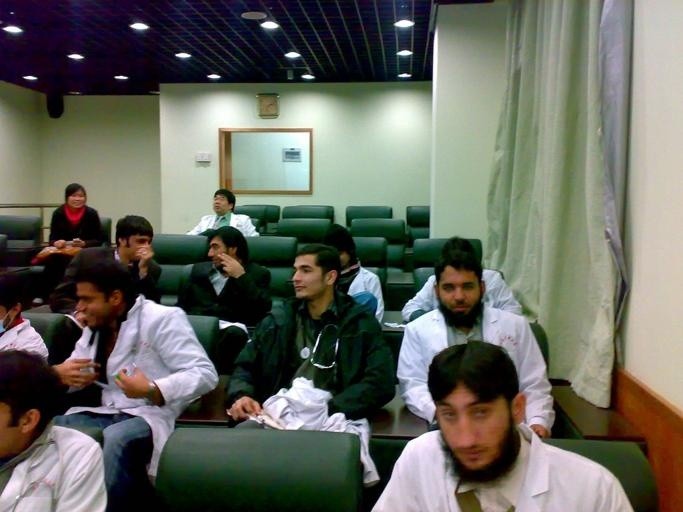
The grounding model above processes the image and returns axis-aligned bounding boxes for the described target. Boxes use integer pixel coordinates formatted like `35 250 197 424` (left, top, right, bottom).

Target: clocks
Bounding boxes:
257 93 282 119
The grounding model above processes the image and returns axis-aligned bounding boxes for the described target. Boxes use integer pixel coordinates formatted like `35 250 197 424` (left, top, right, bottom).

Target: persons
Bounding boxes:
0 350 107 511
50 262 218 494
75 217 161 304
366 341 634 512
402 238 523 324
1 280 48 364
31 183 104 308
396 259 556 437
184 189 259 240
176 226 272 374
318 225 385 323
226 244 395 431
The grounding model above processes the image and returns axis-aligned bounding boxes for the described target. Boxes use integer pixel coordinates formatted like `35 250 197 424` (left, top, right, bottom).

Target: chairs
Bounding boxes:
0 206 666 511
